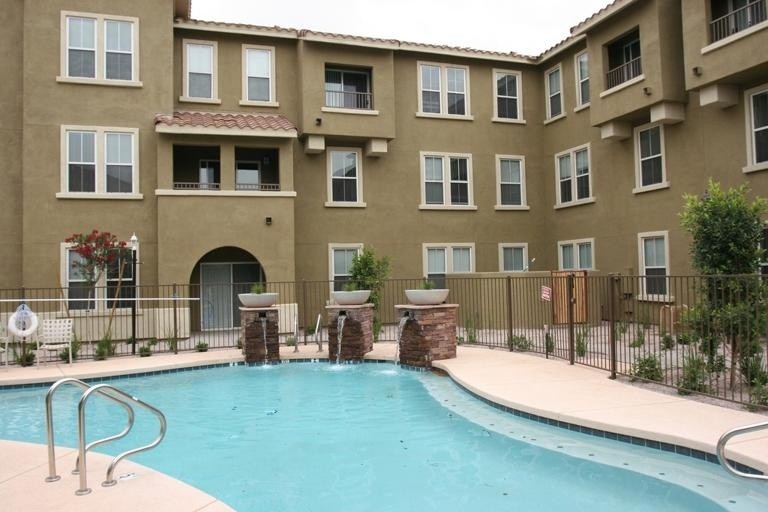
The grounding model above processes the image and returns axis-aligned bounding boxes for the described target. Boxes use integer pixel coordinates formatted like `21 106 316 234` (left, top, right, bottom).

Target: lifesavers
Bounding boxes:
9 311 37 337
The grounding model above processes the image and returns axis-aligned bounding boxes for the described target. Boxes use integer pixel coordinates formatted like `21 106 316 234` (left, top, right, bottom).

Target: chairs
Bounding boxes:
0 336 10 367
35 317 74 366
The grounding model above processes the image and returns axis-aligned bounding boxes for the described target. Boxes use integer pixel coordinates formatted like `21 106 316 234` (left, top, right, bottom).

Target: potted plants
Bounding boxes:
332 281 372 305
236 282 278 306
406 280 450 304
